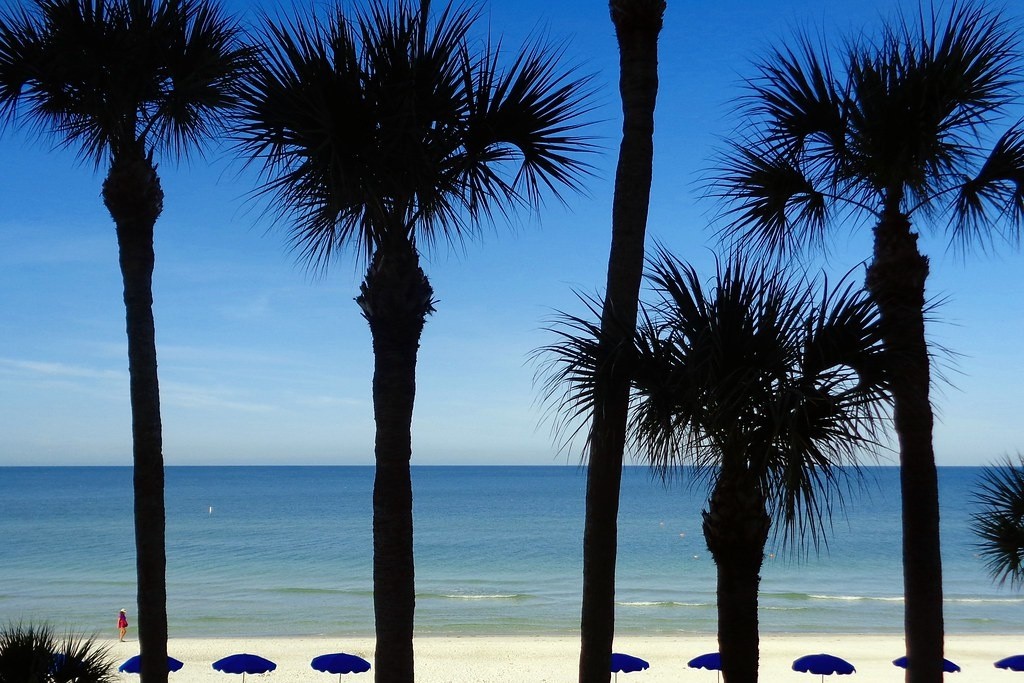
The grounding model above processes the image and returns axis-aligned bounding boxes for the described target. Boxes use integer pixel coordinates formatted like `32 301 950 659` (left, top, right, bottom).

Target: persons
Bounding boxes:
118 608 129 642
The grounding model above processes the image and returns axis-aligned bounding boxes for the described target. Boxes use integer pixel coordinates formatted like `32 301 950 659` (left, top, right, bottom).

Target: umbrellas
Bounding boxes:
212 653 277 683
995 655 1024 672
792 653 856 683
48 654 84 675
892 654 961 673
311 651 371 683
609 653 649 683
688 652 723 683
119 654 184 674
